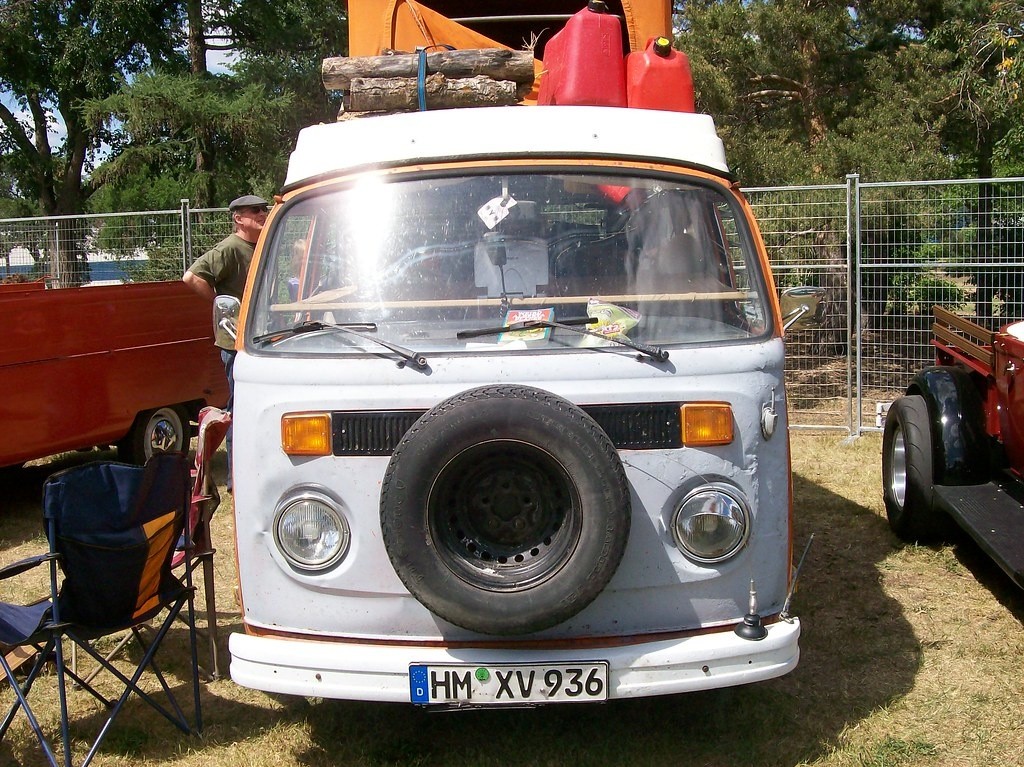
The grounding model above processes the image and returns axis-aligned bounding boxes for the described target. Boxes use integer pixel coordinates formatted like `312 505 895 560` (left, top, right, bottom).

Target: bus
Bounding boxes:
213 104 829 713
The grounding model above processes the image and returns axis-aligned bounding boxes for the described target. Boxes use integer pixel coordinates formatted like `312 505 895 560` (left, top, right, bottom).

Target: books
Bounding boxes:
497 307 555 347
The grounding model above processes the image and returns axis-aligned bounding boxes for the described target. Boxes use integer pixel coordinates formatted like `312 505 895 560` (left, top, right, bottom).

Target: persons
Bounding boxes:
183 195 270 494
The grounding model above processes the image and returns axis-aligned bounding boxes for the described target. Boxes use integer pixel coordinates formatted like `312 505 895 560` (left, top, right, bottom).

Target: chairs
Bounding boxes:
70 406 232 692
0 450 204 767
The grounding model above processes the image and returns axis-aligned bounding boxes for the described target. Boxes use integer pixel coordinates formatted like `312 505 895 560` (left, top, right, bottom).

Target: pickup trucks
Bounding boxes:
0 274 230 480
882 303 1024 592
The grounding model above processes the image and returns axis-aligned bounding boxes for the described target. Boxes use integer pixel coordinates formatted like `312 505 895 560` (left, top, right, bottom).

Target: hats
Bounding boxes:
229 194 268 211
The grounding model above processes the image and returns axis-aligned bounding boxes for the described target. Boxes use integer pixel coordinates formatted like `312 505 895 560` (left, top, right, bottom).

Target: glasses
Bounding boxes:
240 205 268 214
